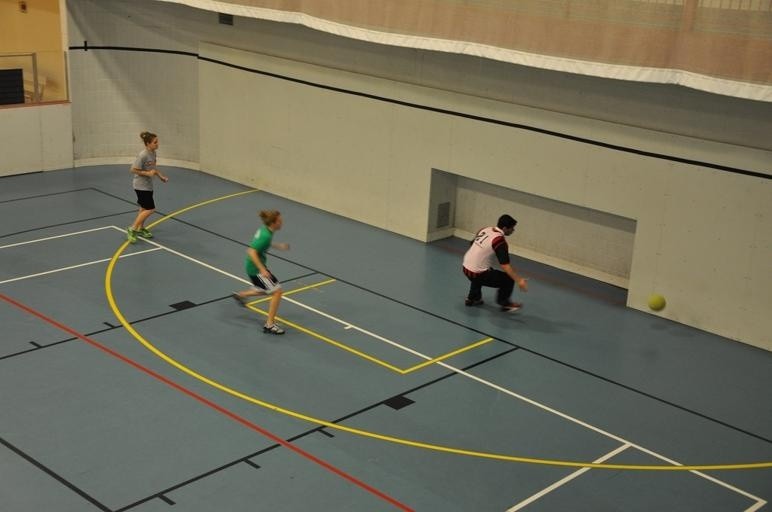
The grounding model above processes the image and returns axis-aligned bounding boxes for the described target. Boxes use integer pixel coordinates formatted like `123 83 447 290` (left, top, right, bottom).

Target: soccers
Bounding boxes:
648 294 665 310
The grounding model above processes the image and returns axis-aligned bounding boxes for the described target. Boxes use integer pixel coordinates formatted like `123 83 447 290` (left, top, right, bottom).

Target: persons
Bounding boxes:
461 214 531 314
230 207 293 335
124 128 169 245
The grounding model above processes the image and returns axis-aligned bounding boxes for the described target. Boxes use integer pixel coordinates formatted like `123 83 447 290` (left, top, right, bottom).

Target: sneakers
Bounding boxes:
263 322 285 334
136 226 153 238
464 296 484 306
500 302 522 314
126 223 138 244
